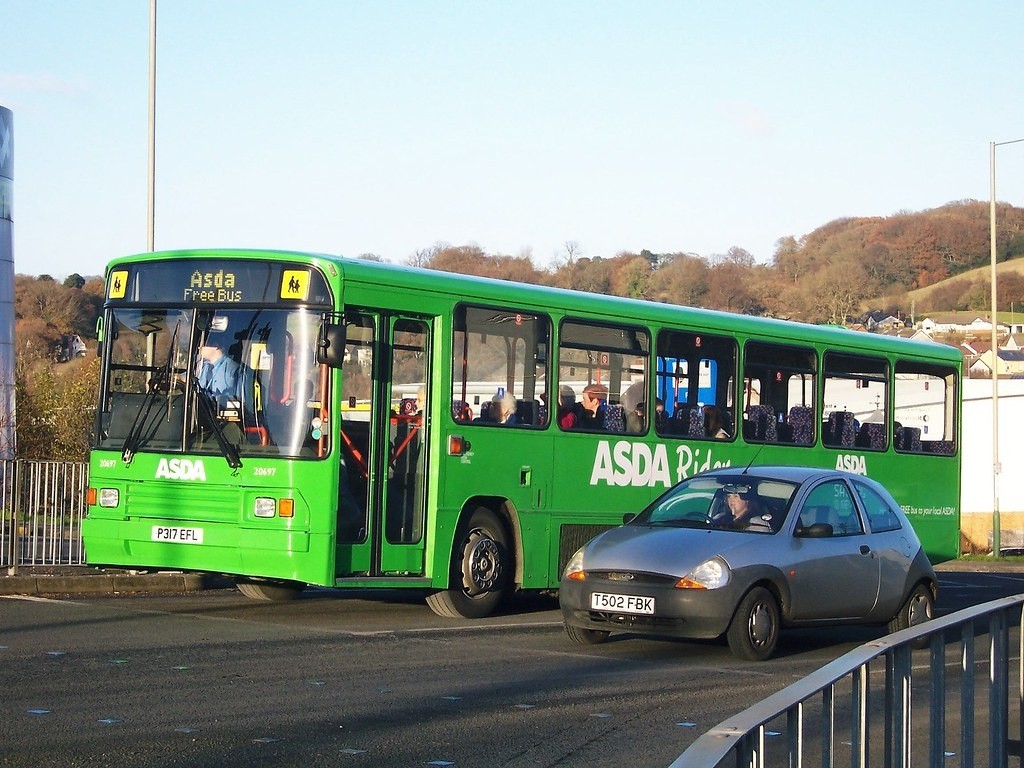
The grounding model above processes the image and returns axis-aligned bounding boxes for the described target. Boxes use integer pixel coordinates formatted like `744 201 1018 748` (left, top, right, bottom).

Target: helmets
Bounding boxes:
723 483 754 502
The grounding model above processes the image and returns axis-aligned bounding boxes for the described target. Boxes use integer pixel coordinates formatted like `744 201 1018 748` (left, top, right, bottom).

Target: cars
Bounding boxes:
558 465 939 663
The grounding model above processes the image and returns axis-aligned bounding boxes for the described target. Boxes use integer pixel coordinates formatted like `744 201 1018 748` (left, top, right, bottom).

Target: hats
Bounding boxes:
583 383 608 400
559 384 575 396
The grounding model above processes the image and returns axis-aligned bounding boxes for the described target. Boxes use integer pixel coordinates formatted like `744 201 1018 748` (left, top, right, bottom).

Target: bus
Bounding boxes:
83 251 965 621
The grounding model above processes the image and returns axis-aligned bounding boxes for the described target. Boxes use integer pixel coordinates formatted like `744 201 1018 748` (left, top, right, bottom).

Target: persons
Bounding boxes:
456 391 523 425
558 384 608 429
702 405 729 438
146 330 262 433
706 479 773 532
286 379 366 536
627 398 673 436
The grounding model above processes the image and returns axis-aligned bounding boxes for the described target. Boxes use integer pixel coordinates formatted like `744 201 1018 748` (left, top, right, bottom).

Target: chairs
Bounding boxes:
824 411 855 446
802 506 841 534
858 422 884 448
923 441 954 453
747 404 777 442
676 408 705 437
895 422 922 452
452 399 474 420
790 406 813 444
516 399 538 424
604 405 626 432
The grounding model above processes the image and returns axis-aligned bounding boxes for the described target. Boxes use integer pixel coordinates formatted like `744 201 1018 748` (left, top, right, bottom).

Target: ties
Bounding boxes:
206 364 214 394
